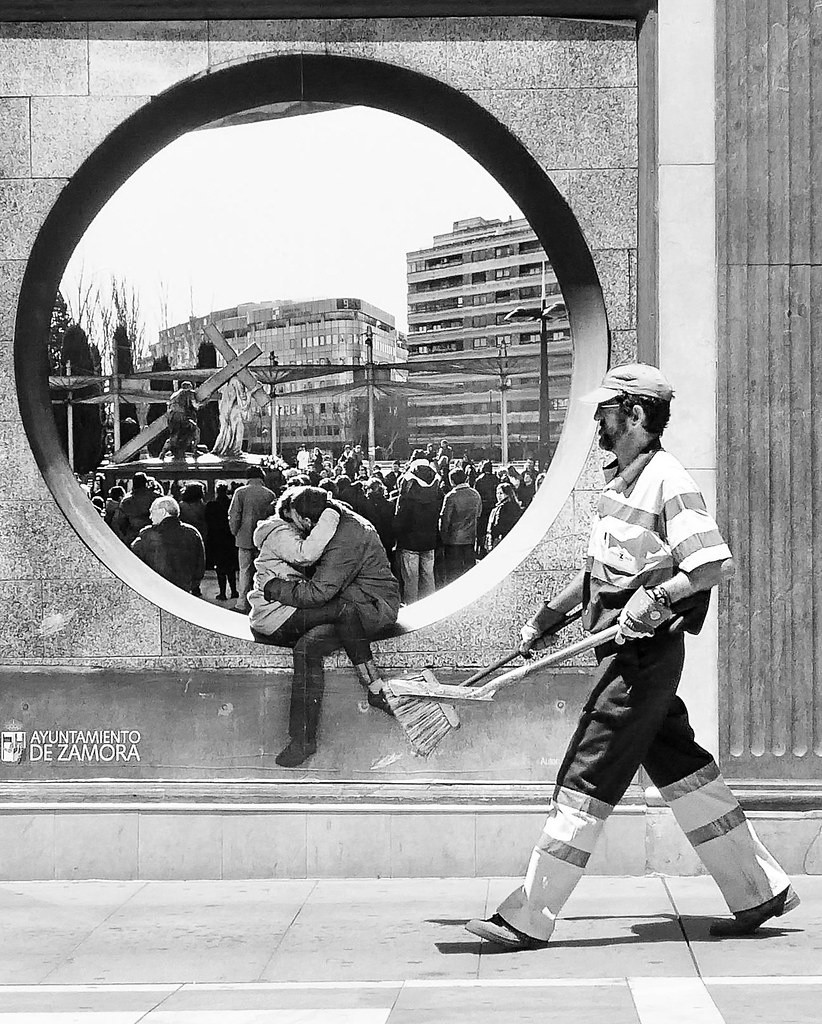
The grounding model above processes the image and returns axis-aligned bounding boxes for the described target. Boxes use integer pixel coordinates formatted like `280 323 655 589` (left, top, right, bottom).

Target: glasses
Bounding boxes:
597 402 627 410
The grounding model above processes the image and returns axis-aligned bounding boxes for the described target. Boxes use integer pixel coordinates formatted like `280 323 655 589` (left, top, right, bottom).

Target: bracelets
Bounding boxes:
655 585 672 606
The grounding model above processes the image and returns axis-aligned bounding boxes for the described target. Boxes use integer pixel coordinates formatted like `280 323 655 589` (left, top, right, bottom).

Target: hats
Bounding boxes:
246 467 266 478
577 362 673 403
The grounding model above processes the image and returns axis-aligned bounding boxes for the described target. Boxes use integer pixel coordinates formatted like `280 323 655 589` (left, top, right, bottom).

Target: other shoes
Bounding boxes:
368 690 395 715
215 593 227 601
231 591 239 598
464 914 550 950
710 885 800 935
274 738 319 768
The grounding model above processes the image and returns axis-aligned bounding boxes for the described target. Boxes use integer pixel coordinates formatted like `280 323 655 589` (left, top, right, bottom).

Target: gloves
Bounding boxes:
518 601 566 657
615 585 672 638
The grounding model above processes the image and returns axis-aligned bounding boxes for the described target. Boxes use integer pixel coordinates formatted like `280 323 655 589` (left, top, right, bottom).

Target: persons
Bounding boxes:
159 381 204 461
464 365 801 949
212 376 264 456
75 443 546 614
248 485 401 767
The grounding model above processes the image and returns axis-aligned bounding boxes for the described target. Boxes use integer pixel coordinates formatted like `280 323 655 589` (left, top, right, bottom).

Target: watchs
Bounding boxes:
650 587 668 609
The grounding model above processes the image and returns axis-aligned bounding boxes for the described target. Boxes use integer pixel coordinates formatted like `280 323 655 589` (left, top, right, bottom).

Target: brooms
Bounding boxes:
381 607 584 759
